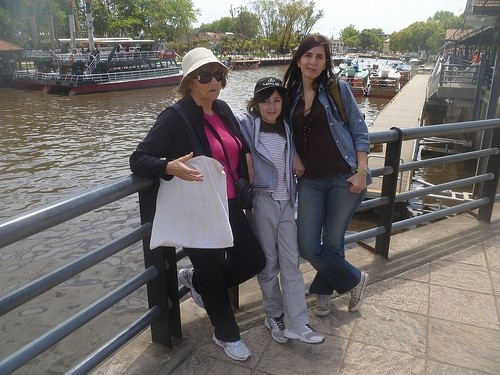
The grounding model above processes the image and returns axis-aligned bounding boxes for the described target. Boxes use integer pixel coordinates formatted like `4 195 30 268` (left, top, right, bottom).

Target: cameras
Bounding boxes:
235 178 253 201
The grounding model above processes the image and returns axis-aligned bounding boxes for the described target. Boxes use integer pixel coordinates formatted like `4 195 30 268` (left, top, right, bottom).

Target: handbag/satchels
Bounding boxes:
235 177 254 208
149 104 234 250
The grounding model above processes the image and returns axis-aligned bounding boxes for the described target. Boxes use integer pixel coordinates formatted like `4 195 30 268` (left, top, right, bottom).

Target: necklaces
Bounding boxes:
302 87 315 153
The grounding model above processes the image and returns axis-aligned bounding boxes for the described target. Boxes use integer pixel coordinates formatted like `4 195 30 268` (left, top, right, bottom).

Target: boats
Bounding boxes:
332 55 422 98
0 0 183 95
228 58 261 68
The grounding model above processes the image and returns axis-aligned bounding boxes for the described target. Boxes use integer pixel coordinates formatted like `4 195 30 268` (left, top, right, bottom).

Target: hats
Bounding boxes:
180 47 230 88
254 77 283 94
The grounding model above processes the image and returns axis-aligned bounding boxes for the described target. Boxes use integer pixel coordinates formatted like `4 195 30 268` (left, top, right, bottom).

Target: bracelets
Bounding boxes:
356 169 367 176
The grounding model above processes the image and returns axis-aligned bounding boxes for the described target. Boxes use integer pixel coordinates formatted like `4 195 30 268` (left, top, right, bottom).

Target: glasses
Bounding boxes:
190 70 225 84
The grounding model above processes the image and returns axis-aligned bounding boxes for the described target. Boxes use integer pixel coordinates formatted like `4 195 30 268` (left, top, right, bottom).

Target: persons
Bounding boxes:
129 47 267 361
0 43 121 81
466 51 480 64
282 34 372 316
233 77 326 345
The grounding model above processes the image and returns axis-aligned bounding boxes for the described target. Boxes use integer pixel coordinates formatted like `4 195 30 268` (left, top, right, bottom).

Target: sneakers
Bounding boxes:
212 331 252 361
284 324 325 345
264 312 288 343
178 268 204 307
348 271 369 311
314 294 332 316
